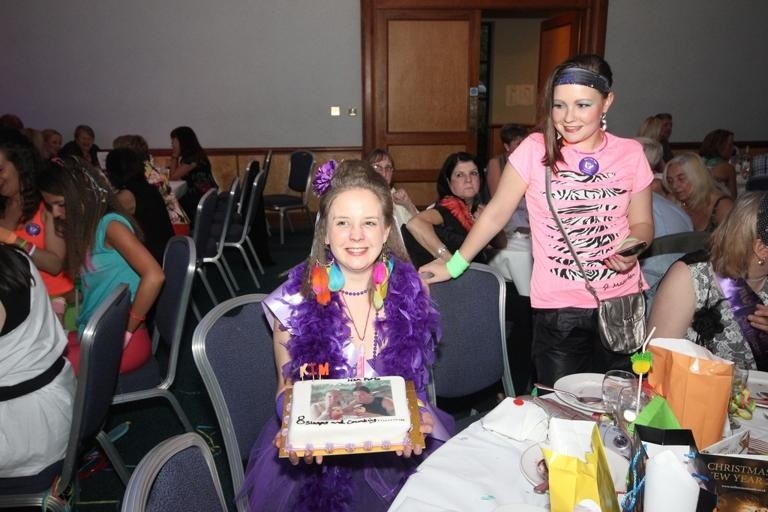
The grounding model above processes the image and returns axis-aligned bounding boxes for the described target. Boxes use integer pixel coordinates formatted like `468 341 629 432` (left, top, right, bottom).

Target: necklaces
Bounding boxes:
338 288 372 355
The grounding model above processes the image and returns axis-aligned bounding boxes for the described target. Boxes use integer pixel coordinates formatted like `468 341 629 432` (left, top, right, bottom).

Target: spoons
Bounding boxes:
533 382 603 405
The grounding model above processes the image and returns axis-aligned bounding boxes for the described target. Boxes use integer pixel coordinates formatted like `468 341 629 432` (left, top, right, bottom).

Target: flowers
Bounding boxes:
309 155 337 197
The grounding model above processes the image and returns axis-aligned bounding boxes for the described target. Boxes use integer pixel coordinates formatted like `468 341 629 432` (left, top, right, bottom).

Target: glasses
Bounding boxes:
374 165 395 174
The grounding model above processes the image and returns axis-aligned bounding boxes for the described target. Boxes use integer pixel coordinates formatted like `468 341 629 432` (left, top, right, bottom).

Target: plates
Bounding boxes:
739 369 768 408
522 440 635 497
554 372 632 414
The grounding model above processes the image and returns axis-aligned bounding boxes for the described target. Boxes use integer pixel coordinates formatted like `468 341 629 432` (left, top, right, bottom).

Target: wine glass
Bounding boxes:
601 371 655 454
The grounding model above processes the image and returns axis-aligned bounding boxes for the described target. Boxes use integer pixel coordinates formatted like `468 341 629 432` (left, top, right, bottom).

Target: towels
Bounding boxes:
484 396 549 446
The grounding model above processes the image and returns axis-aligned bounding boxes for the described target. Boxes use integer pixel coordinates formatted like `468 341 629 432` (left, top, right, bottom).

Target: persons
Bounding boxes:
1 113 215 512
367 129 533 392
636 112 768 371
309 385 392 421
412 54 656 396
235 159 454 512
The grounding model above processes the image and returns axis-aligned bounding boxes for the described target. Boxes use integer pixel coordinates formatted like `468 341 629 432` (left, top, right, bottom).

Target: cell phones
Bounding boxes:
603 242 647 265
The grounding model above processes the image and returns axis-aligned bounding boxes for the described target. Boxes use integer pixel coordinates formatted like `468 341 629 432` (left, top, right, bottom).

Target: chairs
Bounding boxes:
411 261 518 434
639 233 712 314
397 223 487 269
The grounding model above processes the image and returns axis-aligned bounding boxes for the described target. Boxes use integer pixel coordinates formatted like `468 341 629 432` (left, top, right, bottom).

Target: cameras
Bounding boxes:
598 402 632 461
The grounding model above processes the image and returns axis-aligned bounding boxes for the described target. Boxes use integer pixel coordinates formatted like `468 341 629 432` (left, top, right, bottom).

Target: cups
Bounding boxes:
720 354 748 401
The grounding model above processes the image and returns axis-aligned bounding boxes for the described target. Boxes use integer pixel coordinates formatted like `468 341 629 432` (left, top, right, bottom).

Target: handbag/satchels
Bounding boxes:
622 423 716 512
598 292 646 355
